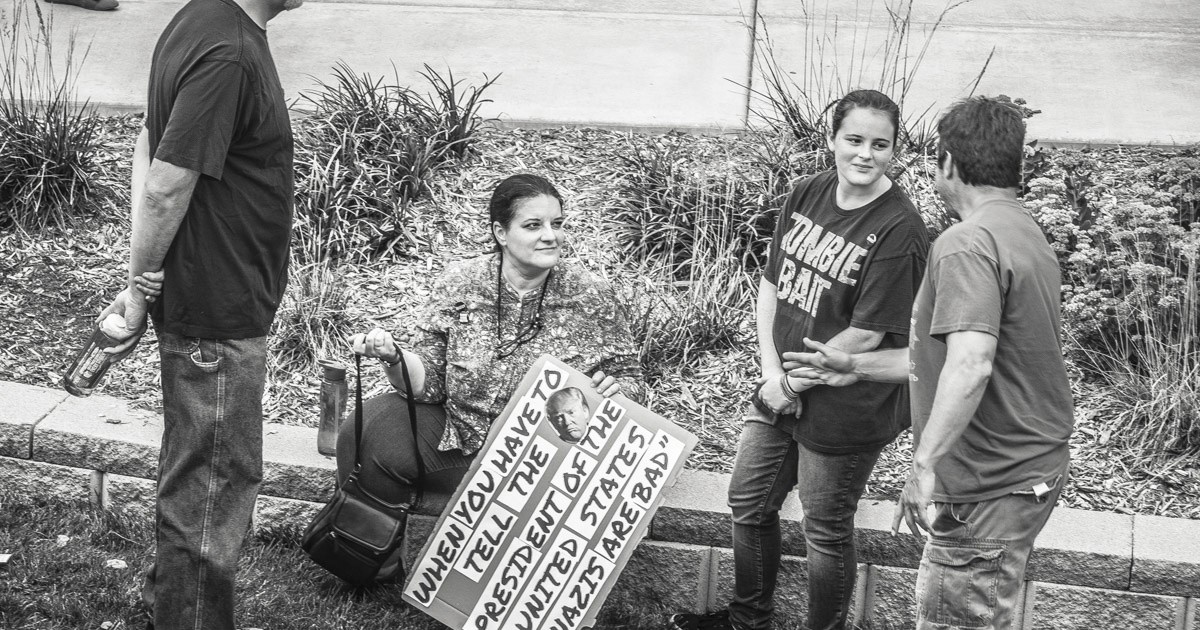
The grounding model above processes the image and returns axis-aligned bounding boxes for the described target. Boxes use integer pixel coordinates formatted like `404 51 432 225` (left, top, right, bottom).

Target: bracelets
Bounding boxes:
780 372 802 404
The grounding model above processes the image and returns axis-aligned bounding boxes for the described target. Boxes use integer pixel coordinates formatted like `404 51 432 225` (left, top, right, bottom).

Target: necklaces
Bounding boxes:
487 251 552 363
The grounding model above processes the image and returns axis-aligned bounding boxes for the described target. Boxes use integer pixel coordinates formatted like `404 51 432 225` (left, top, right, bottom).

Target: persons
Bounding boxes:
546 388 591 446
664 87 928 628
334 172 641 593
94 0 307 630
783 95 1073 630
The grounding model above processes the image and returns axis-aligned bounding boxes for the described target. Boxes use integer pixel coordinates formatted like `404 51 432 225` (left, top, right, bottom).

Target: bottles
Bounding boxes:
60 314 128 399
315 359 349 456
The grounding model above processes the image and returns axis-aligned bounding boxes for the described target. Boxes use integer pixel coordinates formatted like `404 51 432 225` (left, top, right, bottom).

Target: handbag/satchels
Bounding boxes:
301 342 425 586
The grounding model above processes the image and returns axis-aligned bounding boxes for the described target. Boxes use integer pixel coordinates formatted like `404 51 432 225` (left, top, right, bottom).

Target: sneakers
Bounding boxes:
670 609 736 630
